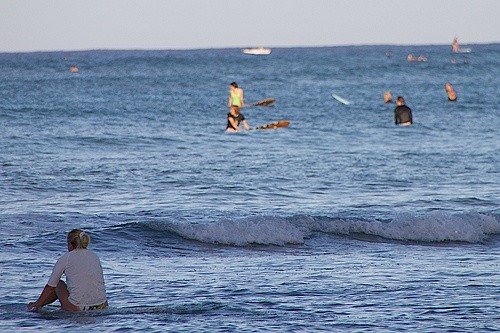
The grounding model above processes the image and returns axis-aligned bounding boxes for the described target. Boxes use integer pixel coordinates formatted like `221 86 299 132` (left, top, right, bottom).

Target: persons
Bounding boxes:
227 82 245 108
393 96 415 125
226 106 250 133
27 228 108 313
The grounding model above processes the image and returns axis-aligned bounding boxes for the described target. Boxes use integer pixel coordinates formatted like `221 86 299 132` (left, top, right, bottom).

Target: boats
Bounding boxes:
243 47 272 55
